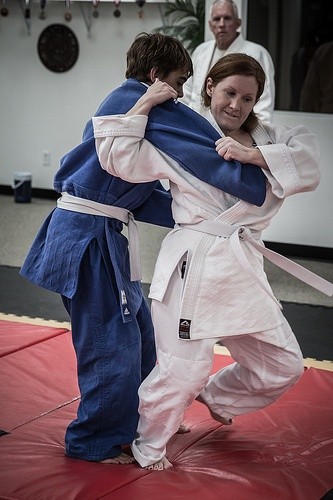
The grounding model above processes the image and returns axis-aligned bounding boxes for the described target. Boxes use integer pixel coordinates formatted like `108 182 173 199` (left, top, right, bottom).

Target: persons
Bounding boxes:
178 0 276 128
17 35 276 464
93 54 321 472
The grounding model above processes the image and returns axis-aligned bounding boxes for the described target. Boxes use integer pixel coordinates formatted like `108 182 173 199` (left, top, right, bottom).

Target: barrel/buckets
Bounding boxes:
11 172 32 204
